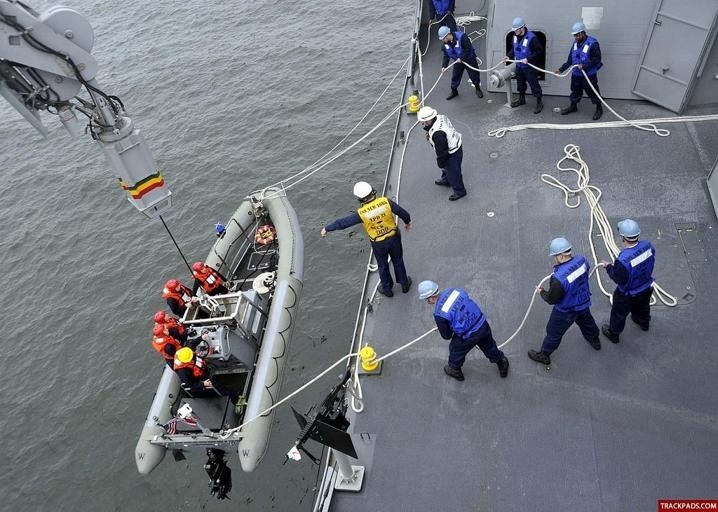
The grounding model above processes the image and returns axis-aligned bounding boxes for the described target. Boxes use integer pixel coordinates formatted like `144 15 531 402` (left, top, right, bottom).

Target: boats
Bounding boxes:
132 178 309 477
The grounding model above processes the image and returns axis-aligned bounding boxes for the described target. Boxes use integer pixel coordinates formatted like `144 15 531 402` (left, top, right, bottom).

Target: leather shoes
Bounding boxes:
435 179 450 187
449 192 467 201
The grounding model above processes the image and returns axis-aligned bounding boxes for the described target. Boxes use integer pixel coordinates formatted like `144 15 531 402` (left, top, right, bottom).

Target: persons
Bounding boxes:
152 262 231 398
321 182 412 296
438 26 483 100
417 106 466 200
555 23 603 120
528 238 601 364
503 17 544 113
602 219 656 343
418 280 509 380
429 0 457 32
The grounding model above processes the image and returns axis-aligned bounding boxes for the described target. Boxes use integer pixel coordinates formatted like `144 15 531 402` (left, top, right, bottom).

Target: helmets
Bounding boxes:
154 311 166 324
353 181 372 198
152 324 164 337
618 219 640 237
512 17 525 31
417 106 437 122
549 238 571 256
418 280 438 299
437 26 450 40
193 262 204 272
166 280 179 292
571 22 586 35
176 347 193 363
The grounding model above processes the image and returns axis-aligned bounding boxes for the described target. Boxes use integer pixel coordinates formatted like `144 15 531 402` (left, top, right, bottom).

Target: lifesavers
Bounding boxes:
254 224 277 245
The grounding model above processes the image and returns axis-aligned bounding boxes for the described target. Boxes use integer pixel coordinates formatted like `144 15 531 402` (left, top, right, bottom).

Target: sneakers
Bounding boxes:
377 285 393 297
402 276 411 293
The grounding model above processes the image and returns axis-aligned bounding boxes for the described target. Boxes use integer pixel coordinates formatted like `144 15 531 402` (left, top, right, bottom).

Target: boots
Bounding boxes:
631 316 649 331
511 92 525 107
447 88 458 100
561 101 577 115
528 350 551 365
587 336 601 349
601 324 619 343
476 84 483 98
593 104 602 120
498 357 509 377
534 97 543 114
444 365 464 381
